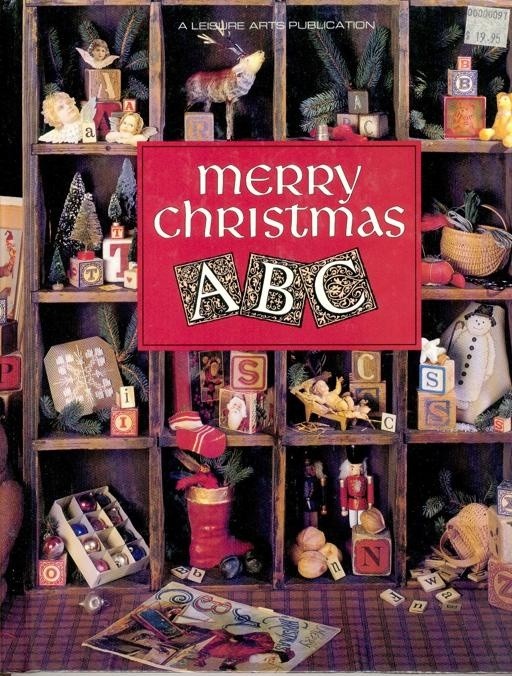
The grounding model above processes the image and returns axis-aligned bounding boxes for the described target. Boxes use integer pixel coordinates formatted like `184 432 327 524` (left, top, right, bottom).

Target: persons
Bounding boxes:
161 622 294 672
75 39 120 70
106 112 159 147
305 376 361 412
37 91 97 145
338 445 375 529
289 446 328 529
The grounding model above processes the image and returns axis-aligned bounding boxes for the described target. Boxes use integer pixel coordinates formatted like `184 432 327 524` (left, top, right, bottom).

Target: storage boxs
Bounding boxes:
48 485 150 588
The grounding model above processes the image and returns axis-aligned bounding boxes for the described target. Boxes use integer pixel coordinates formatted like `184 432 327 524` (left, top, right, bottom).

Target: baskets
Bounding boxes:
440 224 510 275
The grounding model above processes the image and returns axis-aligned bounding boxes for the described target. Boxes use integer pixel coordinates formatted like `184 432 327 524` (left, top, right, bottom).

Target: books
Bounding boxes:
78 579 343 672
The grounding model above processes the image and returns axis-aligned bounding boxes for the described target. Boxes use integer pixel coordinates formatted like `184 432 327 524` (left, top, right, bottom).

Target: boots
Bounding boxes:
187 485 253 570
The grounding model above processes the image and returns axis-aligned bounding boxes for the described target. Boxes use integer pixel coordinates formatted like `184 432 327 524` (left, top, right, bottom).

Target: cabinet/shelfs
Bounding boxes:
26 0 512 597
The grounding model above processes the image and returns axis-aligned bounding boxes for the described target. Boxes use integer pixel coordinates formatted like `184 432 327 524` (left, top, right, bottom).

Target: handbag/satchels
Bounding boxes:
440 502 490 569
